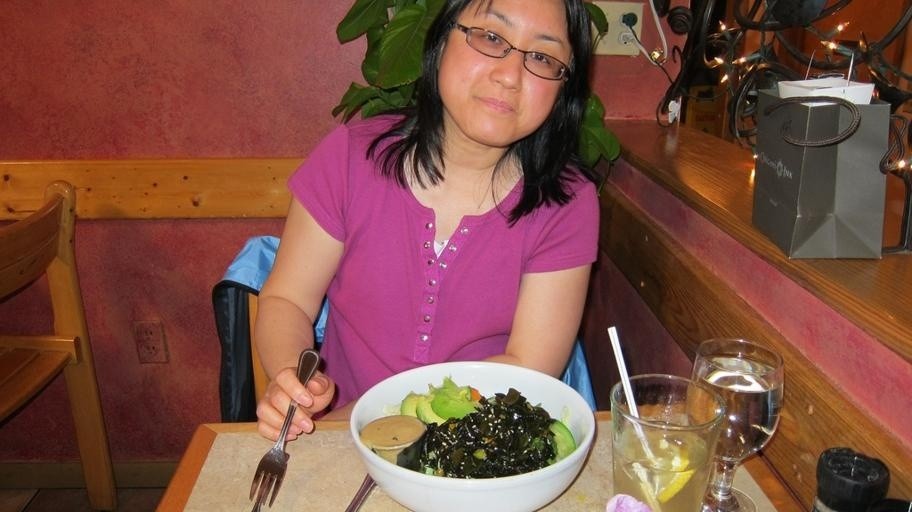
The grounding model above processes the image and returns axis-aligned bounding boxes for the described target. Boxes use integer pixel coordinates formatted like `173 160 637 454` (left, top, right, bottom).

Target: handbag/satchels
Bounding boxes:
749 71 892 264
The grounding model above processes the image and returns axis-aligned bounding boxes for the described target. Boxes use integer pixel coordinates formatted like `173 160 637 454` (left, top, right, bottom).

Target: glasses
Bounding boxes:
441 17 573 86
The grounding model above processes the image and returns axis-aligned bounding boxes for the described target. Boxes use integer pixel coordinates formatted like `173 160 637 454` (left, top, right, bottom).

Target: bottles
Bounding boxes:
811 446 890 512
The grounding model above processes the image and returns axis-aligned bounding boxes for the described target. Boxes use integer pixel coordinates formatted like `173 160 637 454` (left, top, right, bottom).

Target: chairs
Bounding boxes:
1 181 120 510
213 236 593 423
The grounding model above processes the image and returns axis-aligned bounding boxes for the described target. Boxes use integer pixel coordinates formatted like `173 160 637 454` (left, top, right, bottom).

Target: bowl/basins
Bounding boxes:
349 359 597 511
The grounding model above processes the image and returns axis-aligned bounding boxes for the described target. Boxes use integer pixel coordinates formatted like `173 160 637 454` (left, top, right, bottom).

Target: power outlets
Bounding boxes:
589 2 642 56
134 319 167 366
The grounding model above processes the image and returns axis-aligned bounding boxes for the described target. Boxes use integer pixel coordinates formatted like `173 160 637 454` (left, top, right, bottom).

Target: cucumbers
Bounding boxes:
401 374 576 466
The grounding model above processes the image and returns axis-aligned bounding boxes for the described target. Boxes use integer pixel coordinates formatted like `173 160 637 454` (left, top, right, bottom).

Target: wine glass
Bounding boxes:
684 333 784 512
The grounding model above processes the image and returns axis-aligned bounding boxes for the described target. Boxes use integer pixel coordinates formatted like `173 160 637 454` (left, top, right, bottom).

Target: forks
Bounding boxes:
248 348 323 509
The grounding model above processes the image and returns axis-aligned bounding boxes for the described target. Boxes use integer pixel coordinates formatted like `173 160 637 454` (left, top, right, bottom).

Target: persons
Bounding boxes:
252 0 603 442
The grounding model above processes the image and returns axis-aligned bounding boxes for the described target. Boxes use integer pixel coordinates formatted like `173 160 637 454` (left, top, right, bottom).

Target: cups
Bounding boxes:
609 374 727 512
